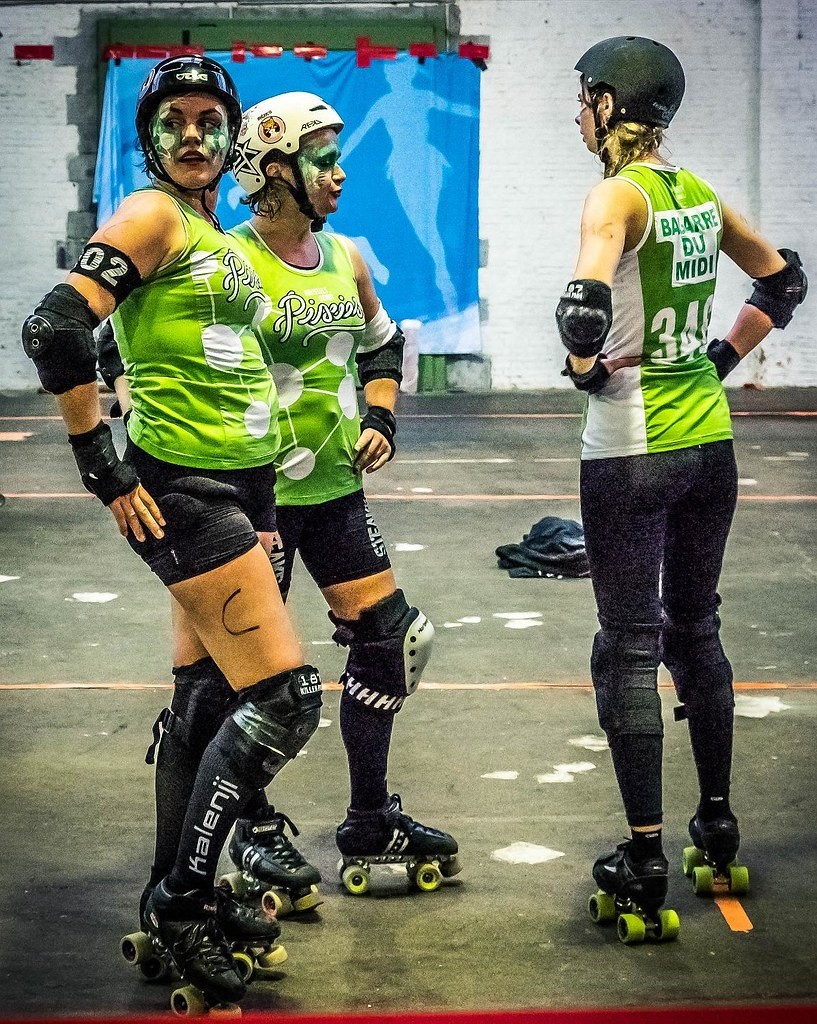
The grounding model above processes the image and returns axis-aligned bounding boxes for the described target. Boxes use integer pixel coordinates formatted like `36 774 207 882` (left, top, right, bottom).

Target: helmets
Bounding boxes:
573 34 686 127
229 90 344 198
135 53 243 159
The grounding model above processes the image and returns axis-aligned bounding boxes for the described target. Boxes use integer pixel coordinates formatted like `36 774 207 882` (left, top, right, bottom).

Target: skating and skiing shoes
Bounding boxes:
120 869 242 1021
334 792 462 893
137 876 288 982
677 804 749 899
587 851 680 944
215 813 324 918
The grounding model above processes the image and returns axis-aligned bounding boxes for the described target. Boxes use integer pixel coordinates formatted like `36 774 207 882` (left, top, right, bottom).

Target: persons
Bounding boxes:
554 35 810 902
22 54 325 987
227 90 461 888
